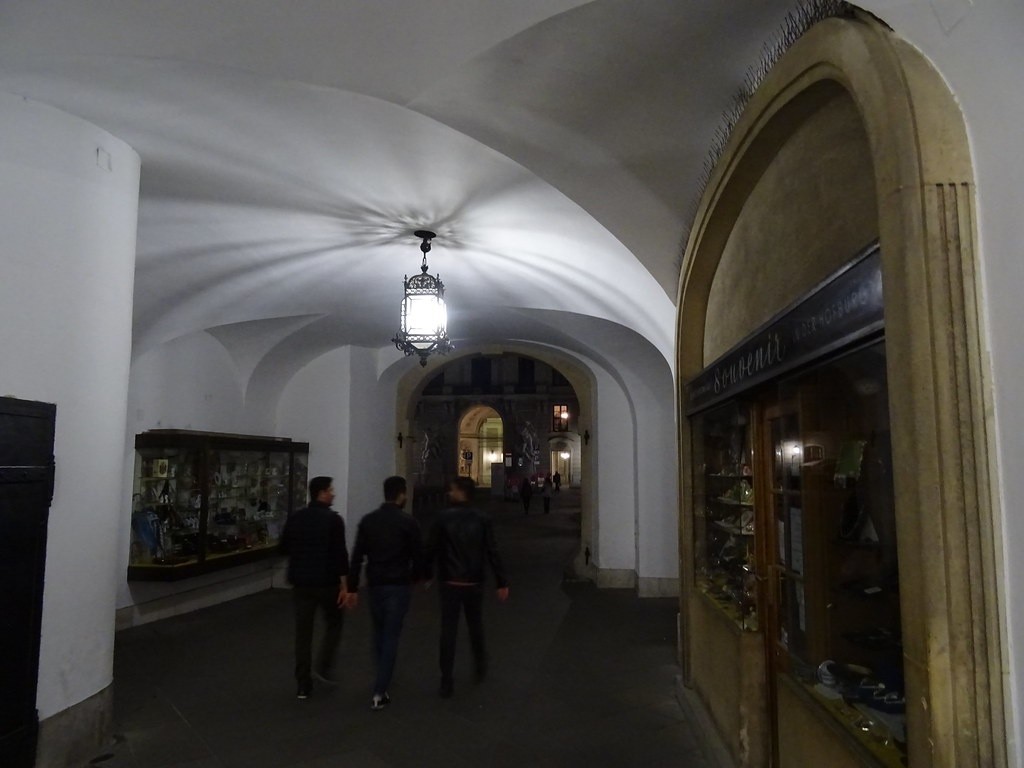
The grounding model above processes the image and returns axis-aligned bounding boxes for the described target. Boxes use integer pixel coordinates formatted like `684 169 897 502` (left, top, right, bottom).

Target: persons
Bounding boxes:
553 471 561 493
346 476 425 709
543 477 552 514
521 478 532 514
425 476 508 696
276 477 349 698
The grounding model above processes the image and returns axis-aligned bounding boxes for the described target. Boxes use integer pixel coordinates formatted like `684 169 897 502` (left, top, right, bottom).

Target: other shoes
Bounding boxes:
296 688 311 699
438 679 453 698
370 687 391 709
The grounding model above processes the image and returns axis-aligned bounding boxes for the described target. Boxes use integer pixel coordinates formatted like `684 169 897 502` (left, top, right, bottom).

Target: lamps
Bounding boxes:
389 231 455 368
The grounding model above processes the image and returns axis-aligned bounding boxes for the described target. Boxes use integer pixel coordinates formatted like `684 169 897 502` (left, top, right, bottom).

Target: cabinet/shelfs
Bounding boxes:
676 343 909 767
129 430 311 581
